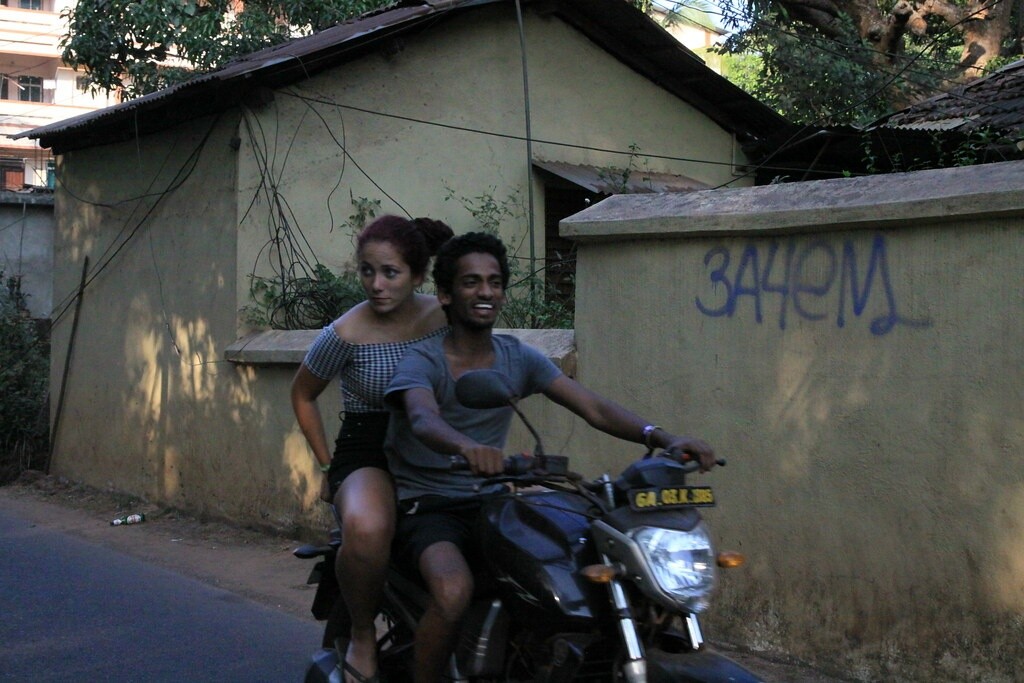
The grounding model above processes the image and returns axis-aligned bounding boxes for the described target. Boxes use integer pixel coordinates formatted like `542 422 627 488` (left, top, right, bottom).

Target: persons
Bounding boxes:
382 233 717 683
291 215 454 683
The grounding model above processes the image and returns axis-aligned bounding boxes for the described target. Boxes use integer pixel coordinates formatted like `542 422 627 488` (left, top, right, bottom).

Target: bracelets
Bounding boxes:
643 424 664 450
319 464 331 472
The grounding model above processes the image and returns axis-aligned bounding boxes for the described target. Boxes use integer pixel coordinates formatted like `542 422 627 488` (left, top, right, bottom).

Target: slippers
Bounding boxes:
341 636 380 683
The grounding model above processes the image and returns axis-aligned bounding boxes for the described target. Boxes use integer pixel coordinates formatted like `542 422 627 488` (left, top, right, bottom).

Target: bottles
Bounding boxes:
110 513 145 526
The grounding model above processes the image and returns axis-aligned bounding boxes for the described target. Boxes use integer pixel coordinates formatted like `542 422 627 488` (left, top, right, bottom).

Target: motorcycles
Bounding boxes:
289 369 779 683
